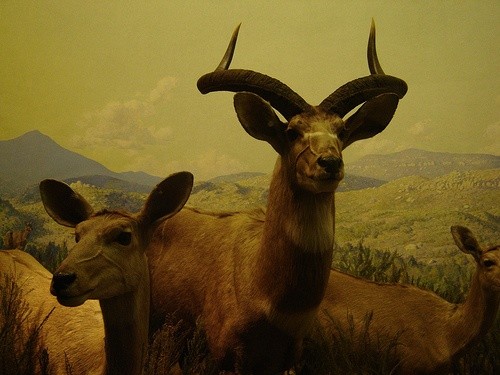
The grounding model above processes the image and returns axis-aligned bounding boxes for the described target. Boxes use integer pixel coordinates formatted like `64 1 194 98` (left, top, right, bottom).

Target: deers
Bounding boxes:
0 19 500 375
